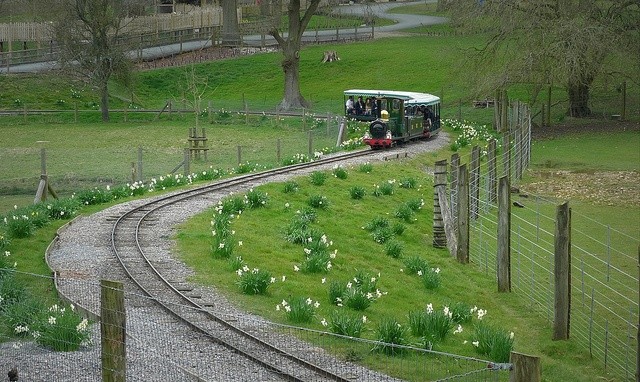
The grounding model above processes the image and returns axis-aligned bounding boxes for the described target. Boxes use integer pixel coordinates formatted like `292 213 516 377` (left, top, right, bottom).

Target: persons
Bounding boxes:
346 96 354 115
353 96 365 115
417 105 435 123
364 98 372 115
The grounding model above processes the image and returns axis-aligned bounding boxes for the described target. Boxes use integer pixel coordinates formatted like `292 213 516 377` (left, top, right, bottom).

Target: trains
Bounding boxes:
343 89 442 149
